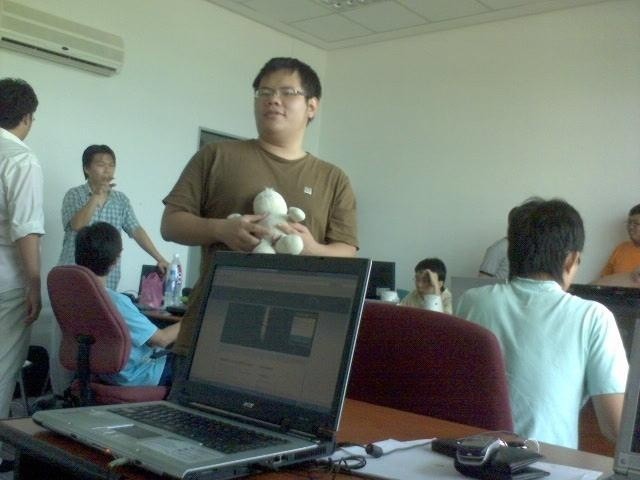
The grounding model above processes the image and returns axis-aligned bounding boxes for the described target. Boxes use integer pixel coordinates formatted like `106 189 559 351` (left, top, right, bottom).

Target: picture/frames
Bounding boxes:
137 264 168 312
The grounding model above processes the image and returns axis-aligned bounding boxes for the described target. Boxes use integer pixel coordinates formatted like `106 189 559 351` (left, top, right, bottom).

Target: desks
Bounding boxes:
2 392 633 480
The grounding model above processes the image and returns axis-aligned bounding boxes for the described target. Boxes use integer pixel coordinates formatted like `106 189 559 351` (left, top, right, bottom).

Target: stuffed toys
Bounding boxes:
227 189 305 255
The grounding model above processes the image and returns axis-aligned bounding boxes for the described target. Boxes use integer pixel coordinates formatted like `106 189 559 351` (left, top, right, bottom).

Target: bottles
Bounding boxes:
164 254 183 308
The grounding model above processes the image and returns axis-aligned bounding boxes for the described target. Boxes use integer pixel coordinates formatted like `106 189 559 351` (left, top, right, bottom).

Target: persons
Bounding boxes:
454 199 630 452
478 233 509 280
52 144 169 400
160 58 360 380
74 222 181 388
400 258 452 314
1 77 46 480
587 203 640 288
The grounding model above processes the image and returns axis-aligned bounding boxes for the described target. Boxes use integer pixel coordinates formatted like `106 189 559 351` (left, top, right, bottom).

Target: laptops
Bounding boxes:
566 284 640 363
602 319 640 480
31 251 372 480
365 261 395 300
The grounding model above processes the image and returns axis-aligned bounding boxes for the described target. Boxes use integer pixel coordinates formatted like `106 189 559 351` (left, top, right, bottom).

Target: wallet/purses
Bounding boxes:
454 446 551 480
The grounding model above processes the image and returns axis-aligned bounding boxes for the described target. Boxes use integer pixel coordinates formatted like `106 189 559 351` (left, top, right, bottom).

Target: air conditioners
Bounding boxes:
2 3 126 76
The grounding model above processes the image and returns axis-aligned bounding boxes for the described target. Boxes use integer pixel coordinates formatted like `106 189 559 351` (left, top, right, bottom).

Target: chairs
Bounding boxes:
43 264 171 401
347 299 514 434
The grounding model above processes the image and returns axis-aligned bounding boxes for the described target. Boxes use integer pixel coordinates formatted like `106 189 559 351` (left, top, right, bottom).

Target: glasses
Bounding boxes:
254 87 310 97
